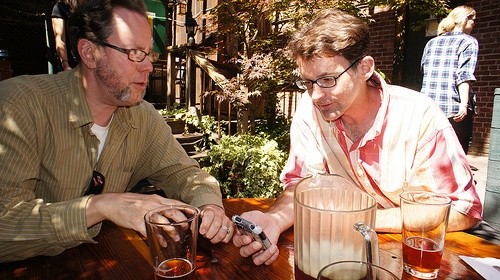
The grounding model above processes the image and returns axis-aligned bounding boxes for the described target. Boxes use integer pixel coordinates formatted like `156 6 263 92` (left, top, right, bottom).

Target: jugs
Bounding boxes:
293 173 403 280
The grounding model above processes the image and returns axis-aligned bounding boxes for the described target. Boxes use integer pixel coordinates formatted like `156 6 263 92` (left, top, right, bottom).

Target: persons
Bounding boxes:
51 0 81 71
233 8 483 266
0 0 235 262
419 5 479 156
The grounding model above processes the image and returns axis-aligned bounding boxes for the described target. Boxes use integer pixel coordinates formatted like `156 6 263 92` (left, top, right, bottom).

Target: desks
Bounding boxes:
0 198 500 280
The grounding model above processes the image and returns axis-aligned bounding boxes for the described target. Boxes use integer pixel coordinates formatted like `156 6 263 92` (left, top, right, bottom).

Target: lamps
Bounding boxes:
425 15 439 37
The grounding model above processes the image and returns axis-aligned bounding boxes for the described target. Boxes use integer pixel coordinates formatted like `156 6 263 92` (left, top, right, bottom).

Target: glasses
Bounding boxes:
83 36 160 63
83 170 105 196
295 54 365 90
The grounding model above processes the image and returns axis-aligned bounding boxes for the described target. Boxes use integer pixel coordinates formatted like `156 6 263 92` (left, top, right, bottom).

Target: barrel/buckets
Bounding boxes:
483 88 500 231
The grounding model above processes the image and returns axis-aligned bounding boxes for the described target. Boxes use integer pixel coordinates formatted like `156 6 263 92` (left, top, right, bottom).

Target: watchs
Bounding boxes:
61 57 68 63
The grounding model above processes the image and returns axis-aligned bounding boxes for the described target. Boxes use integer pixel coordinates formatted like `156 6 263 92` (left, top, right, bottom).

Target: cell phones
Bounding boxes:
231 216 272 250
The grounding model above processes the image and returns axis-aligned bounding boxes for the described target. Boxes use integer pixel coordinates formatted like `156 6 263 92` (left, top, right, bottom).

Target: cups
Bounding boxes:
317 261 399 280
144 205 202 279
378 191 452 280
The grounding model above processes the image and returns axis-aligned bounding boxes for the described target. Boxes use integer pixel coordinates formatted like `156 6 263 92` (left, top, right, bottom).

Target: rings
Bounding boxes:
222 226 230 233
